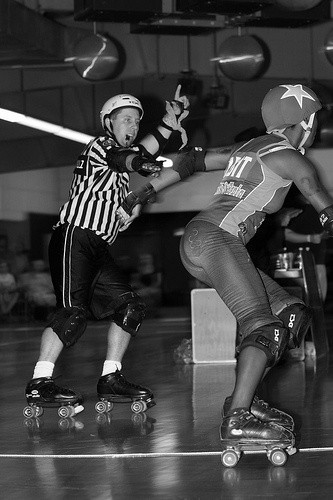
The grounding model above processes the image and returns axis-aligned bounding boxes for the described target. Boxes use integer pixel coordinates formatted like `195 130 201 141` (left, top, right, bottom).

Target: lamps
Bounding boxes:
73 21 120 82
324 28 333 65
217 25 265 81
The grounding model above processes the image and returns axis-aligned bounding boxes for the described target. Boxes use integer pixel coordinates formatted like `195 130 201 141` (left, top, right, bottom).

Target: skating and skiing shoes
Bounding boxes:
220 392 295 432
94 369 156 414
22 376 84 419
219 409 297 468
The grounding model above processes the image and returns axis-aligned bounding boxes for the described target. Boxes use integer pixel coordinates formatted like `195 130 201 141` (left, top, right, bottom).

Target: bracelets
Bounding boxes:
307 235 310 242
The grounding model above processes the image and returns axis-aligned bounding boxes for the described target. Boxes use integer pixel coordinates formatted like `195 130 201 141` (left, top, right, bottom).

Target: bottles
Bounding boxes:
281 247 290 271
297 247 310 269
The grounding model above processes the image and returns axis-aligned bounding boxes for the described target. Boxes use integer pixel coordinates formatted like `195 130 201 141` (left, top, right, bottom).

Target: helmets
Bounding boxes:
260 84 322 132
99 94 144 131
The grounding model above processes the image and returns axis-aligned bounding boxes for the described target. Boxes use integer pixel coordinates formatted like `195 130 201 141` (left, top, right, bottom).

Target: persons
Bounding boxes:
22 253 57 305
115 84 333 467
0 261 20 315
23 84 190 417
285 193 333 356
132 247 162 310
